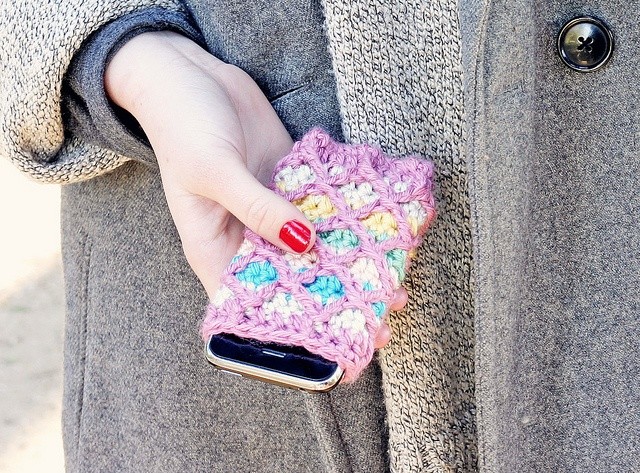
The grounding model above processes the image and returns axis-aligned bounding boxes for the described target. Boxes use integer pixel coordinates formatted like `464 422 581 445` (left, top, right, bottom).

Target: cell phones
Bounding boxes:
205 133 437 394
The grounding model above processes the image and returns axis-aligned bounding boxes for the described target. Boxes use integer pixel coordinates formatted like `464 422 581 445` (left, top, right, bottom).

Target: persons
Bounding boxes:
2 1 639 473
